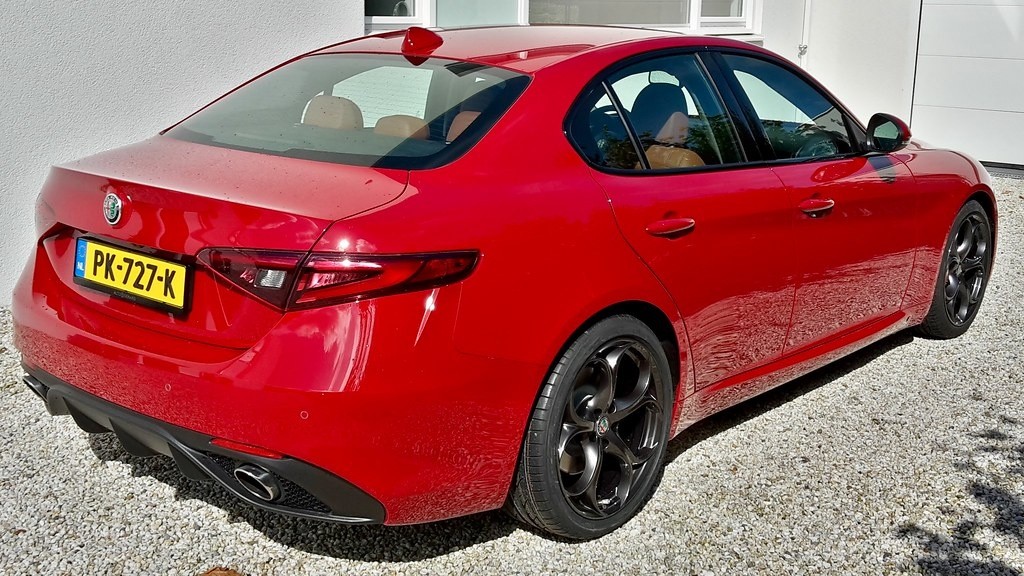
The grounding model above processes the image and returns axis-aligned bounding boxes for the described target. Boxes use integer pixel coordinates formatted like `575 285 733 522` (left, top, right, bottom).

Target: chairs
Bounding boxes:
303 96 364 130
371 113 432 138
626 83 706 168
445 111 483 141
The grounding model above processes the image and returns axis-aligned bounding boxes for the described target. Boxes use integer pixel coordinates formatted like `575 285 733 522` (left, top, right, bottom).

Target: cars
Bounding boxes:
7 22 1001 543
